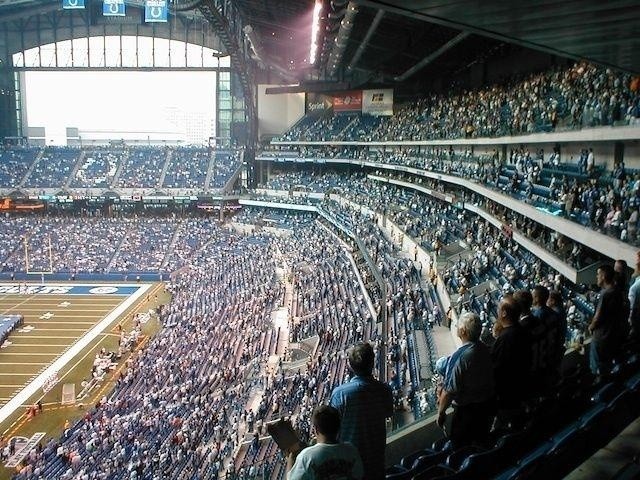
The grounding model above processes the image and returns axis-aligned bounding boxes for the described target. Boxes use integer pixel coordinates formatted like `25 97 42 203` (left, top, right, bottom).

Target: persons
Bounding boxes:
1 140 271 274
277 60 640 146
264 147 639 478
0 228 290 480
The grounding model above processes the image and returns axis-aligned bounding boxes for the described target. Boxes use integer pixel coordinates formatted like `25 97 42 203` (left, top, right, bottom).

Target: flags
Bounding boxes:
145 0 168 23
62 0 86 10
103 0 126 16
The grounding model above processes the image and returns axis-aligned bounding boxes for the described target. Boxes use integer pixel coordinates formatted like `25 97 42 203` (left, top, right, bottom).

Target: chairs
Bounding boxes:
0 76 640 480
